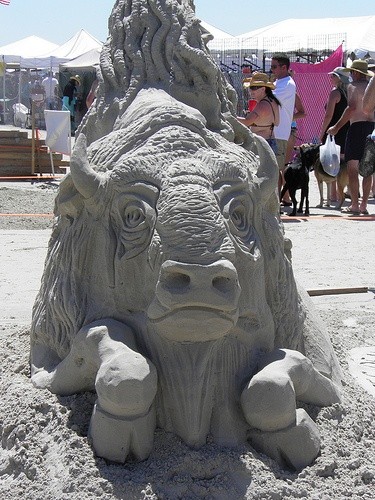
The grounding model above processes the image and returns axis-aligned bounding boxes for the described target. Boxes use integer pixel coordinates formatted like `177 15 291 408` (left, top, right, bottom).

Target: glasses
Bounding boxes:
271 65 280 69
350 70 354 74
250 86 263 91
367 67 375 71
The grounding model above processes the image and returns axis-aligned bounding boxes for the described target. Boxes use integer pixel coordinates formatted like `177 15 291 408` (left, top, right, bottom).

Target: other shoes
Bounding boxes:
344 191 351 198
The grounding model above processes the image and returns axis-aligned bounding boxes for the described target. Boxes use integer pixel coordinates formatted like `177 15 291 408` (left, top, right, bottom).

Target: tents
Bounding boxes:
0 16 375 76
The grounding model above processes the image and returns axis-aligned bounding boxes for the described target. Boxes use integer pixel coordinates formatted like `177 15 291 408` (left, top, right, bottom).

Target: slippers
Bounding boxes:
280 200 292 206
359 209 368 214
341 205 361 214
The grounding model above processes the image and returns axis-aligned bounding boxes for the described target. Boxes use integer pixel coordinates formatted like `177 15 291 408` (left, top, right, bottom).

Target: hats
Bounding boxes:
70 75 80 85
244 73 273 87
328 67 349 83
342 60 374 76
365 58 375 67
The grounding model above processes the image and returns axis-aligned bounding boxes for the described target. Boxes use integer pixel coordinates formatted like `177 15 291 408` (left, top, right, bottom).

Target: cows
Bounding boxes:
27 1 341 471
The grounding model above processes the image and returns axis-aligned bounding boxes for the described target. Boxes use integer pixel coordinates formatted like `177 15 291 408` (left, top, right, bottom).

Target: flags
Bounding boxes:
0 0 10 5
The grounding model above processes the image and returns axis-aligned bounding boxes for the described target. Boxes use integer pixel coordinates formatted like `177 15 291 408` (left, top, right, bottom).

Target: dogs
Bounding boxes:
280 146 318 216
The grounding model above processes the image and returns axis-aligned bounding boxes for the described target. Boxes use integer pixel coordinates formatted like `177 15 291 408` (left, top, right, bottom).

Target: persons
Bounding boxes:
0 71 100 137
234 54 375 216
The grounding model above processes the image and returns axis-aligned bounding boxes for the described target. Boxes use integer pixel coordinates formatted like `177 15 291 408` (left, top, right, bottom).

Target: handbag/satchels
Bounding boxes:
358 136 375 177
320 133 340 176
